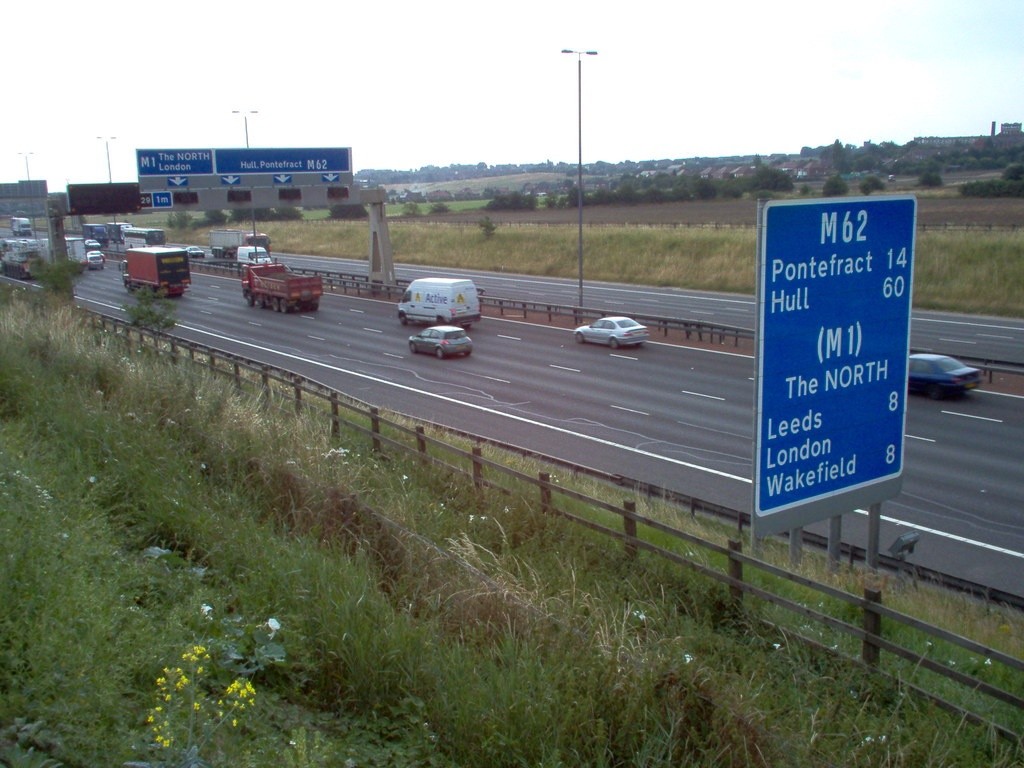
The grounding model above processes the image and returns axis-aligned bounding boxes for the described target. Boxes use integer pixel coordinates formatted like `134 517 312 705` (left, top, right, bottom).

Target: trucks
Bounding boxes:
0 217 87 282
83 224 109 248
107 223 133 244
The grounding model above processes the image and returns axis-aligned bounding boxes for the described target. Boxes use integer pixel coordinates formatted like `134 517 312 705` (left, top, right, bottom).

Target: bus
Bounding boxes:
123 227 166 247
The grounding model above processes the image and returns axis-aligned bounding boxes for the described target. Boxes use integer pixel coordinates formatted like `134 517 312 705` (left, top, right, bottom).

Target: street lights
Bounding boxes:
562 50 598 322
233 110 258 264
18 152 37 240
97 137 120 251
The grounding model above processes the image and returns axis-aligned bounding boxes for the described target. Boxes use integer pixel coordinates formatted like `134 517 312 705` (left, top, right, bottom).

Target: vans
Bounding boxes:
238 246 273 265
398 278 481 330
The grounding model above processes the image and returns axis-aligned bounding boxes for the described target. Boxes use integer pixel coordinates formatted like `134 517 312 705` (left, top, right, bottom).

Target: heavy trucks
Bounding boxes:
209 228 272 259
243 262 324 314
118 248 192 298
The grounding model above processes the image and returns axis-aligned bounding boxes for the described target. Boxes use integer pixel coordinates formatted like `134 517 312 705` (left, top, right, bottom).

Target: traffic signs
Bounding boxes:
136 147 353 191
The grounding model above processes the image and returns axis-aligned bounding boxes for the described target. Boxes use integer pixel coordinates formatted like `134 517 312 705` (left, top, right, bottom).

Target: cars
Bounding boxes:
84 240 101 249
907 354 982 402
409 326 472 360
574 317 649 350
86 251 106 271
186 246 206 258
